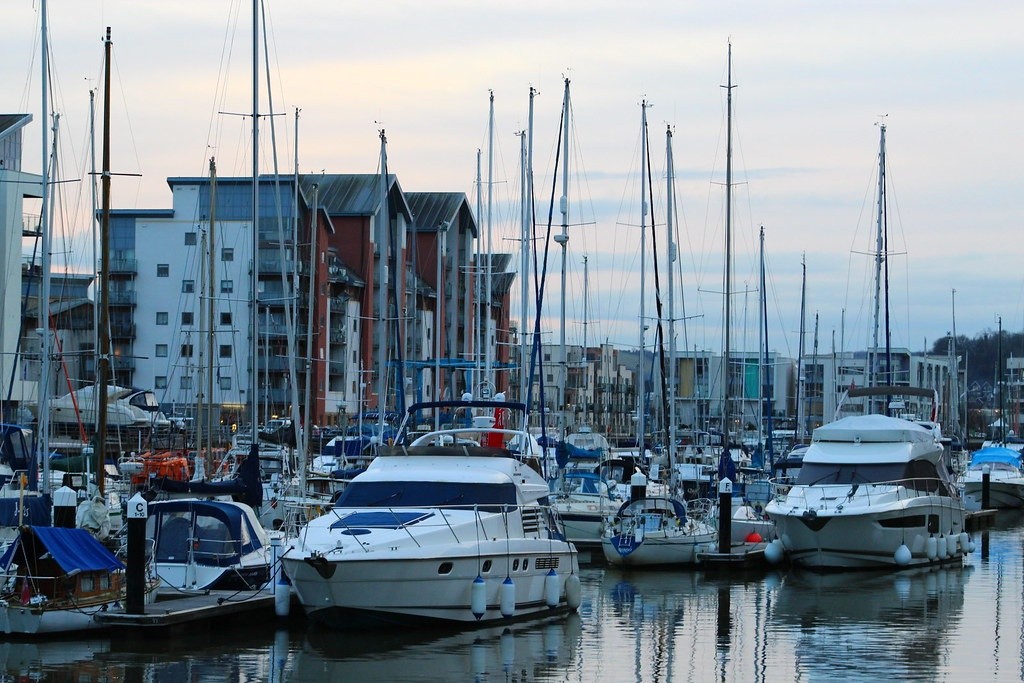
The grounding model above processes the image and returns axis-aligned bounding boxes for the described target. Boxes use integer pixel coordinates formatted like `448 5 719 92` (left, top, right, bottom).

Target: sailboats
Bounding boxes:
0 0 811 553
763 124 975 572
277 148 581 627
956 316 1024 509
599 125 717 568
598 124 716 567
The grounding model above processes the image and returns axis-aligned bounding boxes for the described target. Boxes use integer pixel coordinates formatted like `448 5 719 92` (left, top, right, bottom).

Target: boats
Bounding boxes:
695 475 773 576
0 526 159 638
91 490 302 643
